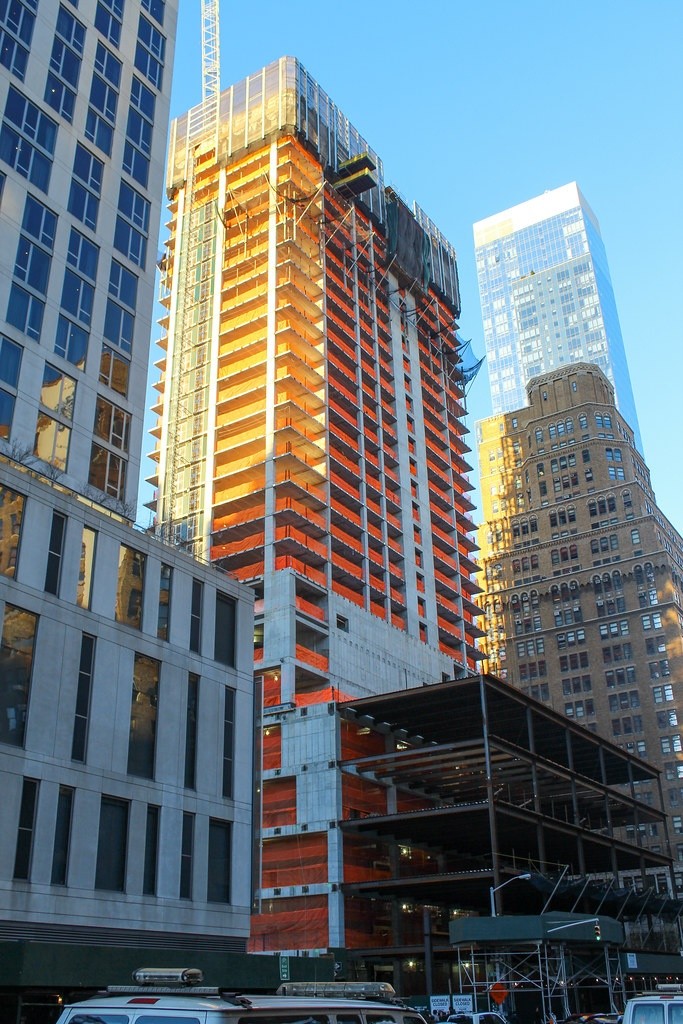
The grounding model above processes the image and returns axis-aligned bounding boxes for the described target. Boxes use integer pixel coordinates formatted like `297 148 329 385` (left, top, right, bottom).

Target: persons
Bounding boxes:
617 1009 624 1024
420 1007 465 1024
534 1007 543 1024
510 1011 520 1024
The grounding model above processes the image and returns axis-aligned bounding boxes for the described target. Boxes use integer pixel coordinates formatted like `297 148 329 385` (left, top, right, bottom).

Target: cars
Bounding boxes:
446 1012 509 1024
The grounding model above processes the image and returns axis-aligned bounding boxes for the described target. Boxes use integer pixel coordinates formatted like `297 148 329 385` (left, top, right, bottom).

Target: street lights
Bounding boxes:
490 873 531 918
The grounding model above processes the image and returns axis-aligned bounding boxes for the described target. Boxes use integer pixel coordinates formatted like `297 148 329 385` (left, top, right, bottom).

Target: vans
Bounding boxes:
622 994 683 1024
56 967 430 1024
